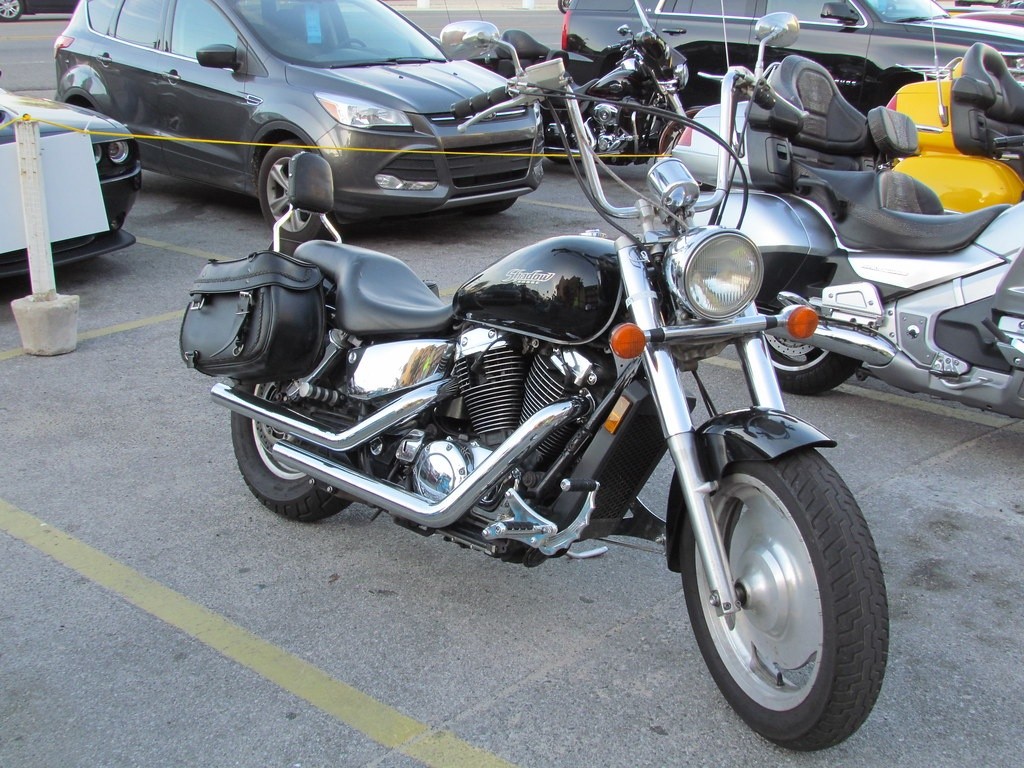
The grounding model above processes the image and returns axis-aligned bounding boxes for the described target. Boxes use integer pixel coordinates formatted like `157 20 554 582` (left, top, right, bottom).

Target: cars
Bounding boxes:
52 0 543 245
0 87 142 278
931 0 1024 28
0 0 79 22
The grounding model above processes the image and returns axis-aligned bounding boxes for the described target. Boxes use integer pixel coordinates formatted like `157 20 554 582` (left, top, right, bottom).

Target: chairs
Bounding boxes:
274 10 307 45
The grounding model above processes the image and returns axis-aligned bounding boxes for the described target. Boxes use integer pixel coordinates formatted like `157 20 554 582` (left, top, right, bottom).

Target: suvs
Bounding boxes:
559 0 1024 122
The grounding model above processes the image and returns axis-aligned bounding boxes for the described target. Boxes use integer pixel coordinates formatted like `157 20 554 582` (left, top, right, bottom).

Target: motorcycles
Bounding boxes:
470 25 717 193
182 13 891 754
669 41 1024 423
